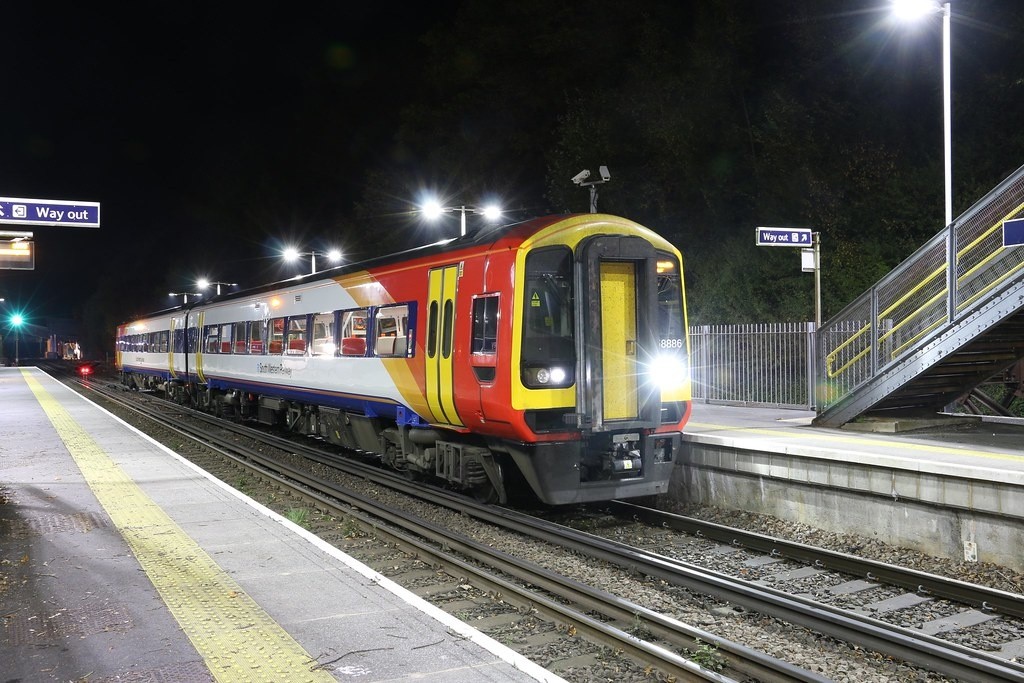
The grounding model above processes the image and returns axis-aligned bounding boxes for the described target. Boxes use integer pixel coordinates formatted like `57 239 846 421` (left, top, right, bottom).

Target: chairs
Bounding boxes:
210 342 217 351
377 337 395 353
342 338 366 355
235 341 245 352
290 340 305 351
269 340 282 353
392 336 406 354
251 341 262 352
222 342 230 352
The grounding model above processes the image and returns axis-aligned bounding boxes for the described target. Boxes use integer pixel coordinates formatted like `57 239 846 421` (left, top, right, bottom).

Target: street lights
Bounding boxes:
10 313 26 363
169 292 202 305
282 247 345 275
423 197 503 237
199 281 239 297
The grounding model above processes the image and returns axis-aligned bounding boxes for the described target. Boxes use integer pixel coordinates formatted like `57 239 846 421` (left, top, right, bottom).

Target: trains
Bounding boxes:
114 210 693 510
